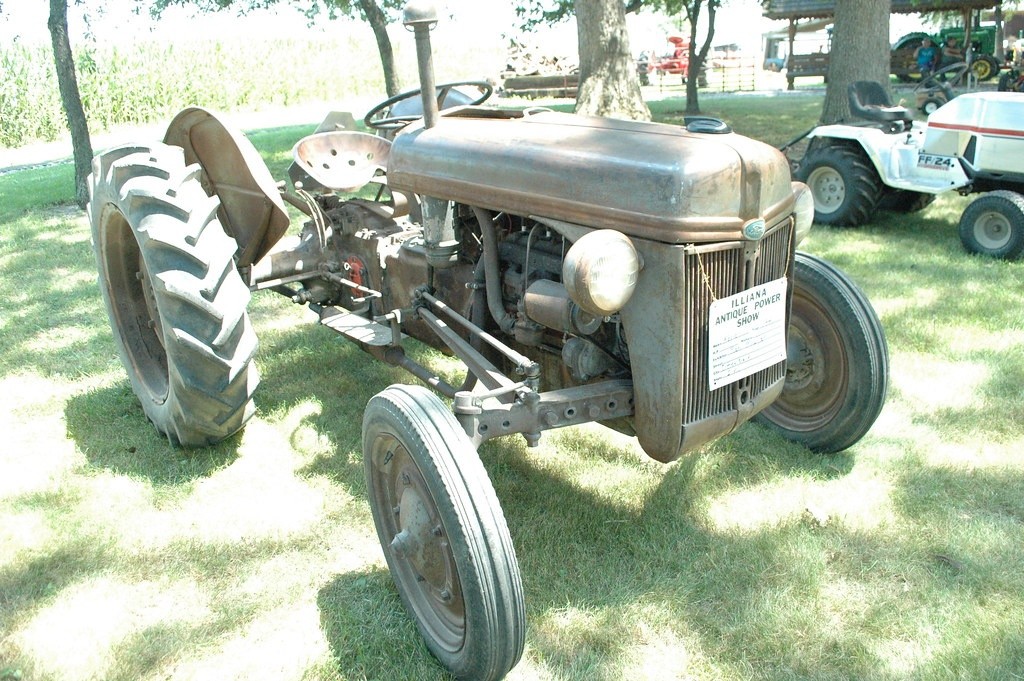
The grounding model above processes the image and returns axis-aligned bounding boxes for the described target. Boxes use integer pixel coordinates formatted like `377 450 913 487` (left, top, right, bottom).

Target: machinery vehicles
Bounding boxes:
893 21 1000 85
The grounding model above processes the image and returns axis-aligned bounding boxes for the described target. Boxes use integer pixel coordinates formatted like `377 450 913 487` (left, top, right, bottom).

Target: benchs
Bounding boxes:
785 51 967 92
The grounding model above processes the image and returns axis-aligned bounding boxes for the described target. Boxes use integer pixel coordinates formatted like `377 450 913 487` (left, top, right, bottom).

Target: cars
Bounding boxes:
792 62 1024 259
84 0 889 681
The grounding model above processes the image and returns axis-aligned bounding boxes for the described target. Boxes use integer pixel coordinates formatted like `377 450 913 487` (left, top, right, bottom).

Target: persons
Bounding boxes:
914 37 938 88
941 35 965 87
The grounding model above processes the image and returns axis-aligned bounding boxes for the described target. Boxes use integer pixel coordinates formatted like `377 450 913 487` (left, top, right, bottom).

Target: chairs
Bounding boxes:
292 127 395 188
847 77 914 131
